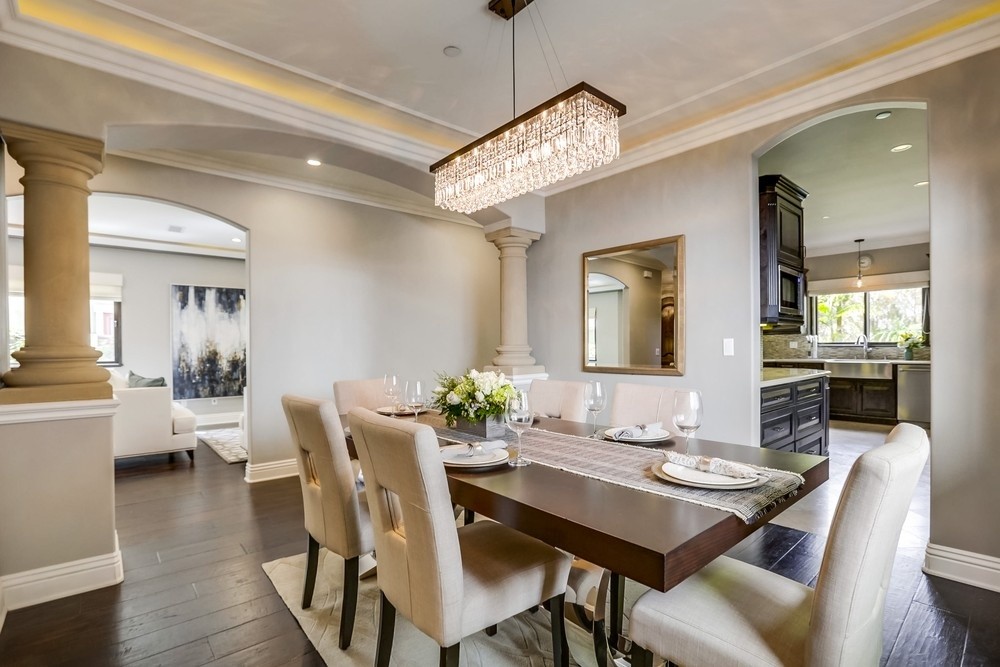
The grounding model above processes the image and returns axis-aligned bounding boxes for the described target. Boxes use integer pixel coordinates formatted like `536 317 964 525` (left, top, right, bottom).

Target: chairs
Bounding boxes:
280 382 933 667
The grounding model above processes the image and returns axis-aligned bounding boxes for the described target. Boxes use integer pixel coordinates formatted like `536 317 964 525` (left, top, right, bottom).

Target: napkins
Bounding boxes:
442 440 507 459
666 451 756 478
613 422 661 440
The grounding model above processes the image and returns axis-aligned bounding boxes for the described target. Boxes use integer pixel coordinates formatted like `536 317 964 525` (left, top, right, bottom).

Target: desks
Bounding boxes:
339 407 829 667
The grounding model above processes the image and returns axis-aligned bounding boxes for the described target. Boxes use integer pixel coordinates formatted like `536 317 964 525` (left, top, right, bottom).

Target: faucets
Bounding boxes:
855 334 872 359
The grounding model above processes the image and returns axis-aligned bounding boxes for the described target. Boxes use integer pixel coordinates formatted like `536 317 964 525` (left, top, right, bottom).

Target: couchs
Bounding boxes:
114 388 197 460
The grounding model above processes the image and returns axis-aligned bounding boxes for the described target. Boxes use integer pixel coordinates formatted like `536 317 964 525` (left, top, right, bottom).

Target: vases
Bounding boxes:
904 348 913 360
456 414 505 438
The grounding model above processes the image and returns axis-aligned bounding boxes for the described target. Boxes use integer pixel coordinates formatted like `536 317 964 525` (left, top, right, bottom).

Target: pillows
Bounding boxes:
128 371 167 388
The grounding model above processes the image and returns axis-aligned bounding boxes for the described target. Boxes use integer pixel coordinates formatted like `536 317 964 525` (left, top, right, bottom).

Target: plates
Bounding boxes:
603 433 672 446
439 445 509 465
377 406 427 415
650 461 769 490
662 463 758 486
444 456 509 473
605 427 670 441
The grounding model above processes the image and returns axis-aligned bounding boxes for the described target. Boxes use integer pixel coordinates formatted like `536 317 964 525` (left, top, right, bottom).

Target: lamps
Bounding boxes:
429 0 628 215
851 238 866 288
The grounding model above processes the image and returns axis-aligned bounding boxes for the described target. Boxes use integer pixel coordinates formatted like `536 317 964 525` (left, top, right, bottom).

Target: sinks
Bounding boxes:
823 358 893 380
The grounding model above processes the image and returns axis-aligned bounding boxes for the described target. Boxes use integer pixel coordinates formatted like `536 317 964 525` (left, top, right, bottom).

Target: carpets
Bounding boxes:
263 511 579 667
197 428 249 463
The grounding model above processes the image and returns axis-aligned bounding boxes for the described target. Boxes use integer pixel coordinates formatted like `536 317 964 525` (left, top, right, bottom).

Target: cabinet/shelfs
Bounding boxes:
762 376 829 457
745 174 809 337
763 362 897 427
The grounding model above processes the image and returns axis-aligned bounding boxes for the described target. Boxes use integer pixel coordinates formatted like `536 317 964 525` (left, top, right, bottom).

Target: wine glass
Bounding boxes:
673 389 704 456
584 380 607 440
505 390 533 467
383 372 402 420
401 379 427 423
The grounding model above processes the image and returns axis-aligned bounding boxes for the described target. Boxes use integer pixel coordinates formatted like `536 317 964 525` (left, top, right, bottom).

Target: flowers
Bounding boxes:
431 367 521 427
897 333 925 350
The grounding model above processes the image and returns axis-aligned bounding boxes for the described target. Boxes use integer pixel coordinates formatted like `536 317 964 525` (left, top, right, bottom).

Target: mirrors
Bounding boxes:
582 234 685 376
